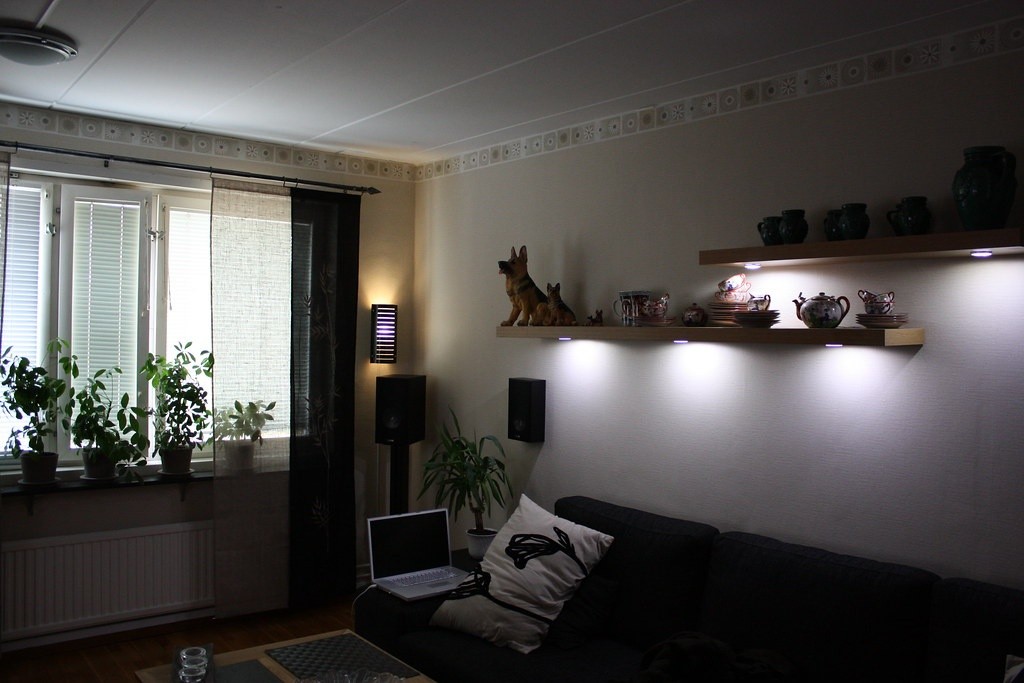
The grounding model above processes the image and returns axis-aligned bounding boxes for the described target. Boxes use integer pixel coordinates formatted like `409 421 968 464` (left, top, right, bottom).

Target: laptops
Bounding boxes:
367 508 482 600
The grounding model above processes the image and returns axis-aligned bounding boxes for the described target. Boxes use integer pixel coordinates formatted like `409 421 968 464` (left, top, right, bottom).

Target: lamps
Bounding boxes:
1 26 79 67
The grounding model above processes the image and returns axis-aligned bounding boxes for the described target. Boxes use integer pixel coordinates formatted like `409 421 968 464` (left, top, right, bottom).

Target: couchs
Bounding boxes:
351 495 1024 682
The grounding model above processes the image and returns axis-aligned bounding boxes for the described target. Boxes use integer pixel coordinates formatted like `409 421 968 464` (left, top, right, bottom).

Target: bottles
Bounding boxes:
822 203 870 242
952 145 1017 235
757 209 809 246
886 195 932 237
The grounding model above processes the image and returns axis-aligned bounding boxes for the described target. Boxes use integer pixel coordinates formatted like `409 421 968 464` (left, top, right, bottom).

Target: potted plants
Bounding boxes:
0 337 276 482
415 405 514 559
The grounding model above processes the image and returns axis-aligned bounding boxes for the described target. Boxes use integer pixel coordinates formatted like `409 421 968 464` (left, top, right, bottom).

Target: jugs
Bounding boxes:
747 294 771 311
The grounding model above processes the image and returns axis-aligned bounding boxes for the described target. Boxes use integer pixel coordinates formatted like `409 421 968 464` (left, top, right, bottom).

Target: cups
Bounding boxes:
681 302 708 327
178 647 207 682
612 290 651 327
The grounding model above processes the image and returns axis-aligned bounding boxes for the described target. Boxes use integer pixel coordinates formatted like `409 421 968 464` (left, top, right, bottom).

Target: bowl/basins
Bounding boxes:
295 668 406 683
713 273 752 305
857 290 895 315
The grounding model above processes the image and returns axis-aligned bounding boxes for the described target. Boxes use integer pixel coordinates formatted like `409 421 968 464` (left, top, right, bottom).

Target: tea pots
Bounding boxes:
792 292 851 329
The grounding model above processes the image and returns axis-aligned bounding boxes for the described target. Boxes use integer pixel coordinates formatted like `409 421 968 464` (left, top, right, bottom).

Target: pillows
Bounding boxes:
428 492 614 653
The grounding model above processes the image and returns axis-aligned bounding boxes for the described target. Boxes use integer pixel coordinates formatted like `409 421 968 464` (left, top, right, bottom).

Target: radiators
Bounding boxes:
0 521 216 651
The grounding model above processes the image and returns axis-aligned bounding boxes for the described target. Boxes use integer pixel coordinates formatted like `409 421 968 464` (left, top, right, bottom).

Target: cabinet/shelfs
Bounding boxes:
495 226 1024 348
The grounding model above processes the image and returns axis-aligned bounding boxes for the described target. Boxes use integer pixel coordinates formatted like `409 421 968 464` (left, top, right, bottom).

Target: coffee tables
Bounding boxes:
134 627 436 683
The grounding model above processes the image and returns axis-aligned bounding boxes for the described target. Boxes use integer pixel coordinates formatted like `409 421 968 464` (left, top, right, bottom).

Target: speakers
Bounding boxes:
376 373 426 445
506 378 545 443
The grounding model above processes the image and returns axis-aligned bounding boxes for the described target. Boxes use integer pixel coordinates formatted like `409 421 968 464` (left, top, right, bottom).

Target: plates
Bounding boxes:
855 312 911 329
706 300 780 328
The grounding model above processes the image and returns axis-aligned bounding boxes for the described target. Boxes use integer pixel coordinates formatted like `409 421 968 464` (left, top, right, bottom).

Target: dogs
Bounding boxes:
497 244 604 326
